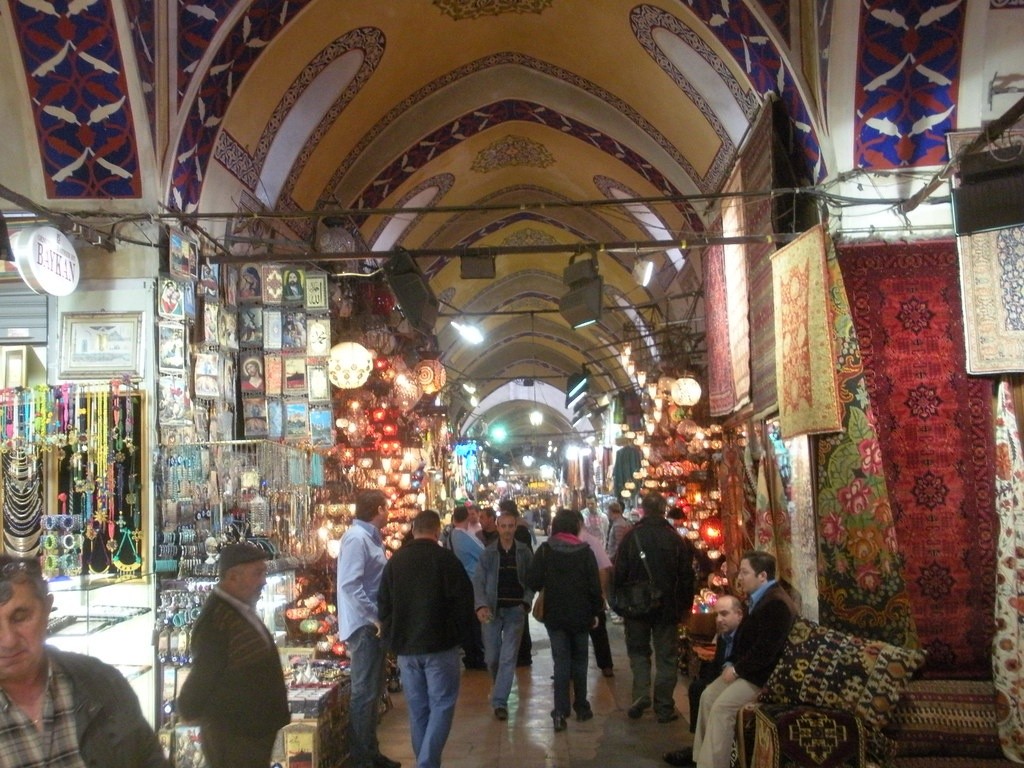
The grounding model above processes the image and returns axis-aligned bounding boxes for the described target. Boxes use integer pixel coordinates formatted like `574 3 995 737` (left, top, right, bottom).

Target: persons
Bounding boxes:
664 595 744 768
474 513 535 718
693 550 798 768
0 553 169 768
527 510 605 730
500 499 533 666
607 503 631 624
540 507 550 536
578 511 613 676
466 506 482 533
578 494 610 548
615 492 693 722
337 493 401 768
667 508 700 559
178 544 291 768
445 506 487 670
378 510 474 768
475 507 499 548
524 504 537 545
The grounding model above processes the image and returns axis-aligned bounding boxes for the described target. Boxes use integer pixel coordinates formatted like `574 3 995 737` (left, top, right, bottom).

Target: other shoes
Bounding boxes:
628 695 652 720
576 710 593 721
657 715 678 723
374 755 401 768
662 745 697 768
602 668 614 678
495 707 508 719
553 716 566 729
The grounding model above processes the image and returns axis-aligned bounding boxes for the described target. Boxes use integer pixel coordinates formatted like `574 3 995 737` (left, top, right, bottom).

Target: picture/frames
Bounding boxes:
58 311 145 382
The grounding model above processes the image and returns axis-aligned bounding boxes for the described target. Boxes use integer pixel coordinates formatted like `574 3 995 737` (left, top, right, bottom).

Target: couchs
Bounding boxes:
737 680 1024 768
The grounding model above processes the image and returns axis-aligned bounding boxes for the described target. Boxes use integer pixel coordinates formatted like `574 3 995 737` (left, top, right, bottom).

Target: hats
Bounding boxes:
219 543 273 575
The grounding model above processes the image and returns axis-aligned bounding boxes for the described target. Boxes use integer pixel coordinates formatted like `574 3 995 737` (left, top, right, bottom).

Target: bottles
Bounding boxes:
294 663 320 683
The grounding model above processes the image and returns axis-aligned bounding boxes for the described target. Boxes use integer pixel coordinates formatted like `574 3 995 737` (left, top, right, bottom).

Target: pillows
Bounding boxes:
753 617 927 732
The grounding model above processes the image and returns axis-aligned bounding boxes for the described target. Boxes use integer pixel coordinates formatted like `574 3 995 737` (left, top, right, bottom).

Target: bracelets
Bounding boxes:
158 588 201 626
160 529 202 571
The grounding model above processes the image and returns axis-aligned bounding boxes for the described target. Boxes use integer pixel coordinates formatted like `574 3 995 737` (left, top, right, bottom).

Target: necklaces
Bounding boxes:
0 373 142 573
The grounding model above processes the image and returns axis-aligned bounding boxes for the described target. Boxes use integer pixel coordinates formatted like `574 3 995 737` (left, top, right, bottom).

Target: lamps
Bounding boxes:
559 247 603 330
524 378 536 386
565 325 708 427
330 245 440 337
631 250 654 286
460 256 497 280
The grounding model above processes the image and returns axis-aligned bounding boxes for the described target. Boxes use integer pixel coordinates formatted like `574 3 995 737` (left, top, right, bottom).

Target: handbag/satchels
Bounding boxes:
615 582 667 620
532 586 545 623
651 588 683 627
